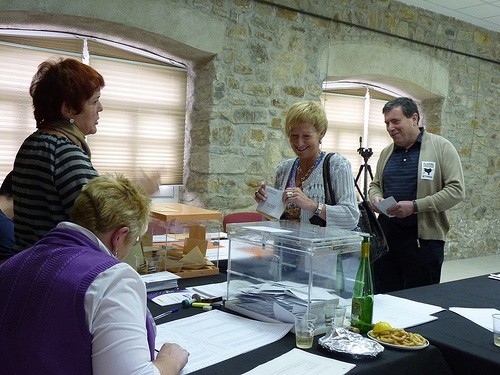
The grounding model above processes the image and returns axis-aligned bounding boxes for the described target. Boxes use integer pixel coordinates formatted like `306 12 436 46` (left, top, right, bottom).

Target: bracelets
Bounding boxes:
313 200 323 217
412 199 418 215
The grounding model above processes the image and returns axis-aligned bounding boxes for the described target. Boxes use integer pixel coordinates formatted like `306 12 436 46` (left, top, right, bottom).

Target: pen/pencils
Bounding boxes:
154 349 159 353
139 242 146 267
153 307 180 321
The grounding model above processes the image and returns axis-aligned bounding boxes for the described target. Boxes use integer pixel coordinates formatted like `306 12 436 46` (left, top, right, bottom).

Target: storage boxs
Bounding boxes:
225 221 370 337
146 202 223 279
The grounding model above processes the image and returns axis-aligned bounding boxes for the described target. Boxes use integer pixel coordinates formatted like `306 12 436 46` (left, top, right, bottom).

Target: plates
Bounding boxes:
367 328 430 350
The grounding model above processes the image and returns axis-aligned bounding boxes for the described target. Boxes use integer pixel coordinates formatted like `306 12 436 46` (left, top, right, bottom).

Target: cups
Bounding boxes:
493 314 500 347
134 252 161 275
294 311 318 349
322 305 346 337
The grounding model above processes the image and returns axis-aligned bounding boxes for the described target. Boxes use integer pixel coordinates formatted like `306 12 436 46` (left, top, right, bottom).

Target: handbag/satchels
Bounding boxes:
309 153 388 274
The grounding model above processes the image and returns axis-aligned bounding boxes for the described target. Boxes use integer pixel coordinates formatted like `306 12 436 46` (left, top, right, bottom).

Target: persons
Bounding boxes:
253 100 360 295
11 57 105 257
367 97 466 296
0 170 190 375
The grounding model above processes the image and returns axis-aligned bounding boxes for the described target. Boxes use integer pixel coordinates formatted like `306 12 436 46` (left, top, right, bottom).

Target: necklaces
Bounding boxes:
296 149 322 182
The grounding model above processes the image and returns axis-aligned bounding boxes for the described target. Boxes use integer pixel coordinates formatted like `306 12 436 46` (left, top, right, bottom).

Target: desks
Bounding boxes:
372 272 500 375
152 233 274 272
150 273 441 375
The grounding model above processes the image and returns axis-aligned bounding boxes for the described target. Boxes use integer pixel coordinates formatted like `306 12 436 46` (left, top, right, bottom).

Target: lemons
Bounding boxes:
373 321 392 333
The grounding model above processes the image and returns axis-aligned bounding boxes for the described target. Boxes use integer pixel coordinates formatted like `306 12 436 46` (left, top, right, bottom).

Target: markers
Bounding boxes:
192 302 221 309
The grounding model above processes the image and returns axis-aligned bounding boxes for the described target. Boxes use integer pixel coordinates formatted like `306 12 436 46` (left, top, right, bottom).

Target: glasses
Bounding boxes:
134 234 141 246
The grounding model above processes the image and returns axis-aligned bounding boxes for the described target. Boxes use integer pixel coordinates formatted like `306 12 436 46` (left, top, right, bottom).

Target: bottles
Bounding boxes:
352 235 374 334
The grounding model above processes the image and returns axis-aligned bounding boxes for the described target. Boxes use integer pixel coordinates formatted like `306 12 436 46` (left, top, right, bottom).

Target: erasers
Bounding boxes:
202 305 212 311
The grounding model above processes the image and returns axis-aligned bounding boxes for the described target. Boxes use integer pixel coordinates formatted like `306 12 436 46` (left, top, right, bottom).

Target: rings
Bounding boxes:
287 192 293 198
254 191 259 195
